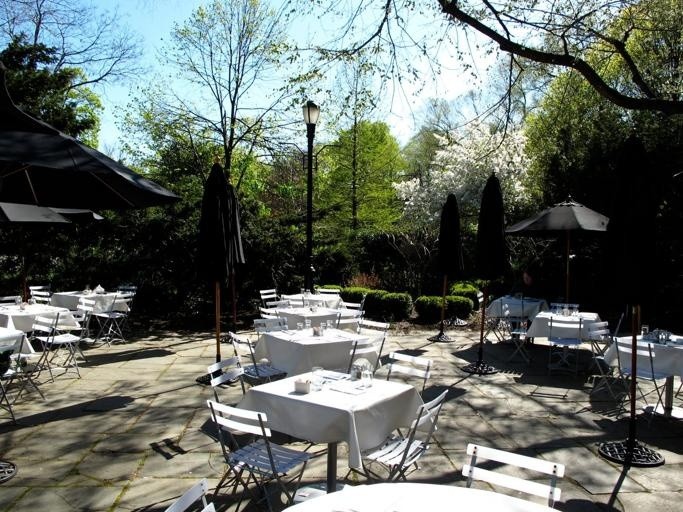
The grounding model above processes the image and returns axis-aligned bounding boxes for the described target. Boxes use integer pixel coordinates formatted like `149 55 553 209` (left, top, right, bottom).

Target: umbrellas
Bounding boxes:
222 178 247 329
471 172 509 361
0 62 182 210
196 159 235 364
432 194 466 332
504 195 632 304
1 203 106 302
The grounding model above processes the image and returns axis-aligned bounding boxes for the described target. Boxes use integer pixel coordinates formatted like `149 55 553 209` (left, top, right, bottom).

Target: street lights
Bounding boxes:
301 99 320 294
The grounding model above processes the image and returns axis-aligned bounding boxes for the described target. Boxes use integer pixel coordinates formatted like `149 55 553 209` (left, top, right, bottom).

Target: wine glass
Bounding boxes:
549 301 582 318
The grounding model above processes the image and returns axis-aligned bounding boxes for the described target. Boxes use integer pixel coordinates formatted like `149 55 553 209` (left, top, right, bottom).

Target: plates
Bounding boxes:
522 297 540 302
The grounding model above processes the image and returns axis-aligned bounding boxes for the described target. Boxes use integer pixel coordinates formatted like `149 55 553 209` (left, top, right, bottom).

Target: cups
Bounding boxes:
296 318 334 342
281 286 327 315
9 295 49 313
638 326 683 346
84 284 107 296
291 360 374 394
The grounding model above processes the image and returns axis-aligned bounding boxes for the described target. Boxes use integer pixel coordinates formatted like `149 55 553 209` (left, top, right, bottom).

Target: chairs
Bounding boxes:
0 283 137 425
476 287 682 421
163 477 221 512
458 442 565 508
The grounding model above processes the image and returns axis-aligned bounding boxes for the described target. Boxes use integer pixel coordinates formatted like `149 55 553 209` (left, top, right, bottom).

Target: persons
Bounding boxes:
511 269 542 343
